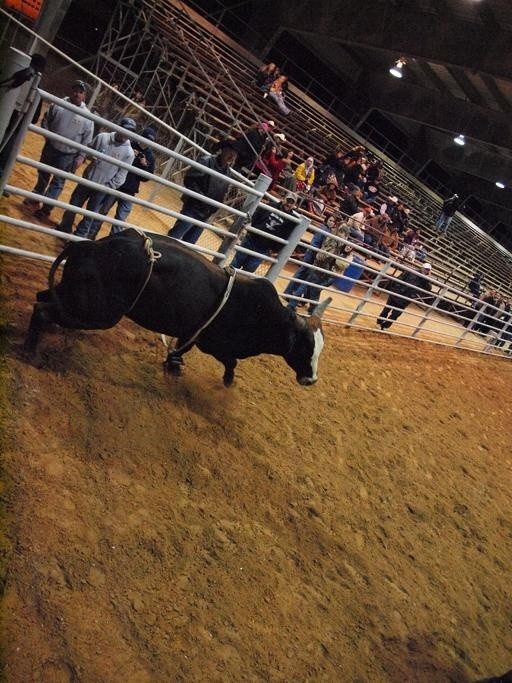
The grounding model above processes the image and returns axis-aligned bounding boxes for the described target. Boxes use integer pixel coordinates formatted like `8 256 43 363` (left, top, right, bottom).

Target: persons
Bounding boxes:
464 289 512 348
88 126 157 240
55 117 138 237
94 83 119 117
255 61 292 117
23 78 96 217
436 196 460 235
120 87 146 119
437 193 457 221
167 139 243 244
231 119 433 331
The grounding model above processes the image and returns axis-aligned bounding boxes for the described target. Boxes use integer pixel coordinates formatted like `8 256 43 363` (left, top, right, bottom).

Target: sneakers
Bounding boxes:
23 197 40 205
282 296 315 312
377 323 387 332
33 209 50 220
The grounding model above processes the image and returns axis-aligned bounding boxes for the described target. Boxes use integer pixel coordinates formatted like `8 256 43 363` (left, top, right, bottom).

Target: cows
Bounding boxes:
21 227 334 390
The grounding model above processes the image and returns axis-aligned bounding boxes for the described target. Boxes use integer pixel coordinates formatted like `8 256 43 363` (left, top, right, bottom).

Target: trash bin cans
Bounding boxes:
335 255 365 293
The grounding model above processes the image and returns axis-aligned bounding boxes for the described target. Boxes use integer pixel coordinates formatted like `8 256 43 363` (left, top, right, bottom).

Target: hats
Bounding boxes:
404 208 410 216
258 120 286 144
218 139 244 153
71 80 87 91
389 195 399 205
326 180 338 189
143 128 155 139
285 193 297 203
382 213 387 219
120 117 137 131
364 207 372 213
422 262 431 270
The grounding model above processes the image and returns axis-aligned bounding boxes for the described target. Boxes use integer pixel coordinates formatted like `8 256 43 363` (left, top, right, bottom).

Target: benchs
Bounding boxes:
147 1 511 320
